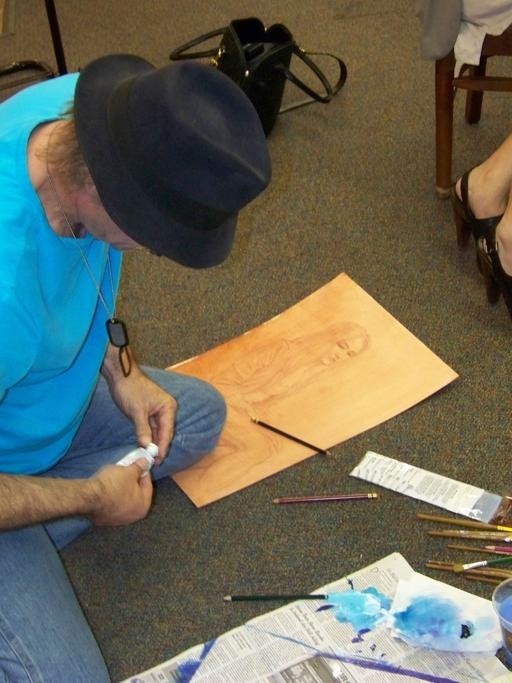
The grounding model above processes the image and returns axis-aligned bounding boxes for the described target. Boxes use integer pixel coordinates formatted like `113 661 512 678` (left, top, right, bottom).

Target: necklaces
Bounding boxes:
44 116 133 377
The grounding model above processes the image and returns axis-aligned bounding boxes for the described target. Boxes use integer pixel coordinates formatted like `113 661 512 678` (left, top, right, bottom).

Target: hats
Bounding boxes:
75 54 272 268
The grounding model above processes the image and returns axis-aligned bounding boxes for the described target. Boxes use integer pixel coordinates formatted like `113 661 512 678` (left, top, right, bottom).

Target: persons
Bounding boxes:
201 322 372 481
2 52 273 683
417 0 512 309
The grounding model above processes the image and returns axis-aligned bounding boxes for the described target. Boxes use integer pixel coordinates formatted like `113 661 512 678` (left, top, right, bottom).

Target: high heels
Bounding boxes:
450 165 506 275
476 233 512 318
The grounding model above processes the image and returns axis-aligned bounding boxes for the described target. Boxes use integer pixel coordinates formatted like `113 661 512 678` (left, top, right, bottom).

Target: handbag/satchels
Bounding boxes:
170 18 347 142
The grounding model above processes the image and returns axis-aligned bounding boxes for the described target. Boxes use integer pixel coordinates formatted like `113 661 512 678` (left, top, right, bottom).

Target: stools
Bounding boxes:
434 16 512 200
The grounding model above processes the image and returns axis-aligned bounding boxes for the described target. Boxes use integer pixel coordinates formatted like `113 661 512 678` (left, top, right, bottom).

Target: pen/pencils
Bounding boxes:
250 417 332 457
270 492 378 504
222 594 328 601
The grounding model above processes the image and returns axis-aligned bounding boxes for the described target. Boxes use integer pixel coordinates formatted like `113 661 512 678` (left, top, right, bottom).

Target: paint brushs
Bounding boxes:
417 514 511 585
